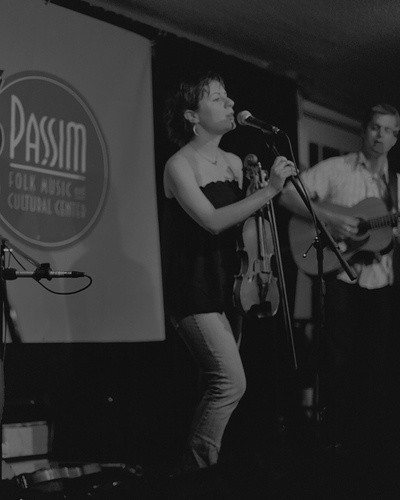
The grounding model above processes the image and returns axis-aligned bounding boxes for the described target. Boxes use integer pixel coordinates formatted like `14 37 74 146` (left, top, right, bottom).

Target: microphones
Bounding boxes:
237 110 287 137
3 267 86 281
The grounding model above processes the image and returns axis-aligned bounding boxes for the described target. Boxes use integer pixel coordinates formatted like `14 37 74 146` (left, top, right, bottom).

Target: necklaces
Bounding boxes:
188 141 218 165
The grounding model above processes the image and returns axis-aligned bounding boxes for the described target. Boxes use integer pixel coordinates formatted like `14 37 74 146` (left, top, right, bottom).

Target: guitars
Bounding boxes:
287 196 400 278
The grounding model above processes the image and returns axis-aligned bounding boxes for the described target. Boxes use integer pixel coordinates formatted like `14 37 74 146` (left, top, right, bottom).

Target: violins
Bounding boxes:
230 154 278 318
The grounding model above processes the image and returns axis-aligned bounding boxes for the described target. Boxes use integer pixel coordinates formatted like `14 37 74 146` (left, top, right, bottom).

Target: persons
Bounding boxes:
278 103 400 434
161 70 300 485
